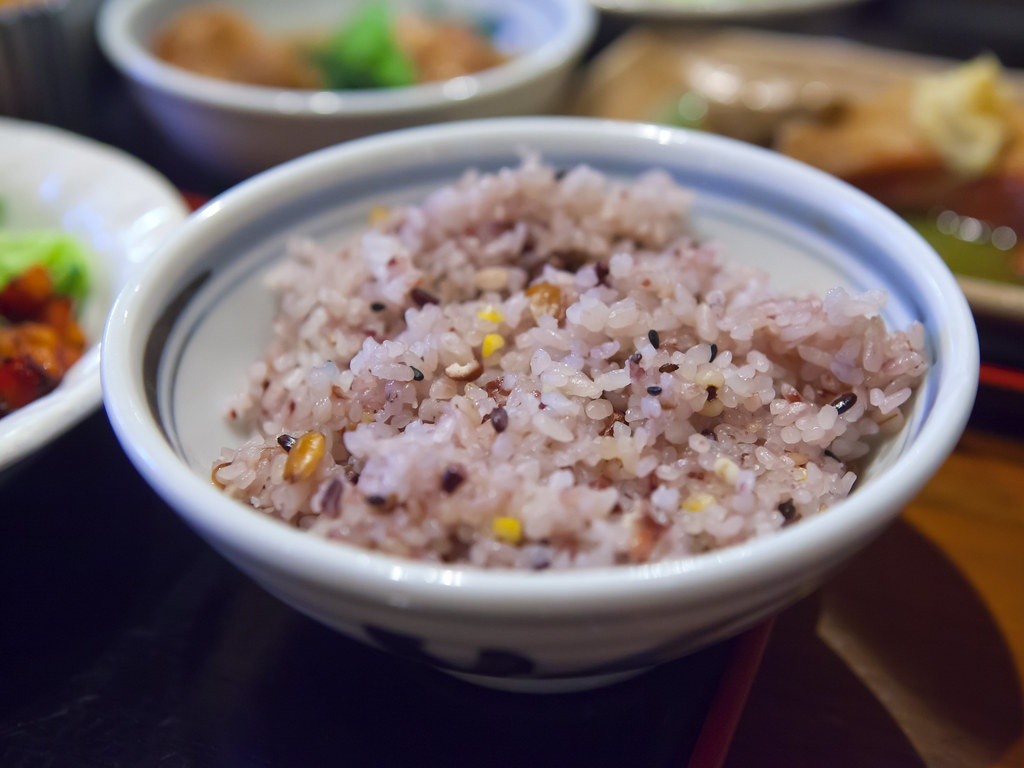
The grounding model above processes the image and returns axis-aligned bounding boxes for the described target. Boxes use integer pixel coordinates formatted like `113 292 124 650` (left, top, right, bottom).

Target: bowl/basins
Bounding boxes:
108 110 982 696
95 2 606 173
2 110 192 489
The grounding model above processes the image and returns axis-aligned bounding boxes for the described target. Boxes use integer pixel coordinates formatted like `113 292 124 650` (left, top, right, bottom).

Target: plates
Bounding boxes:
587 21 1024 399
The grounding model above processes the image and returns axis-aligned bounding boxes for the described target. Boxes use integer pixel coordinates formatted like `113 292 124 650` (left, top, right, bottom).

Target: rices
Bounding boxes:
211 146 927 566
